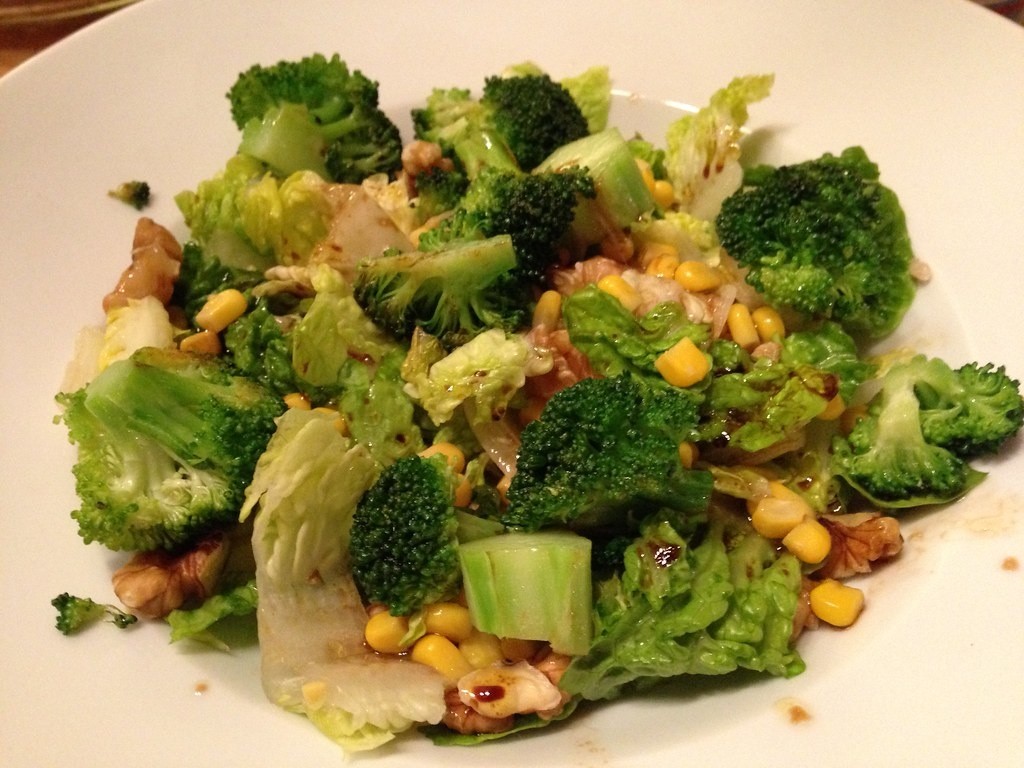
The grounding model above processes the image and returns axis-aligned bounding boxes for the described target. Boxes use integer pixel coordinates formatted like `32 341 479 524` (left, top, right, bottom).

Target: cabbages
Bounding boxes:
176 63 989 753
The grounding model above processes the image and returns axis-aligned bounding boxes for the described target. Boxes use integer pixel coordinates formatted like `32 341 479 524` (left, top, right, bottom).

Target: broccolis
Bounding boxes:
51 52 1024 618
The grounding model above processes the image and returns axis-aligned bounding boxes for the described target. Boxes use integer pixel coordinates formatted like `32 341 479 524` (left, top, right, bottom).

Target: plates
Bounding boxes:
0 0 1024 768
